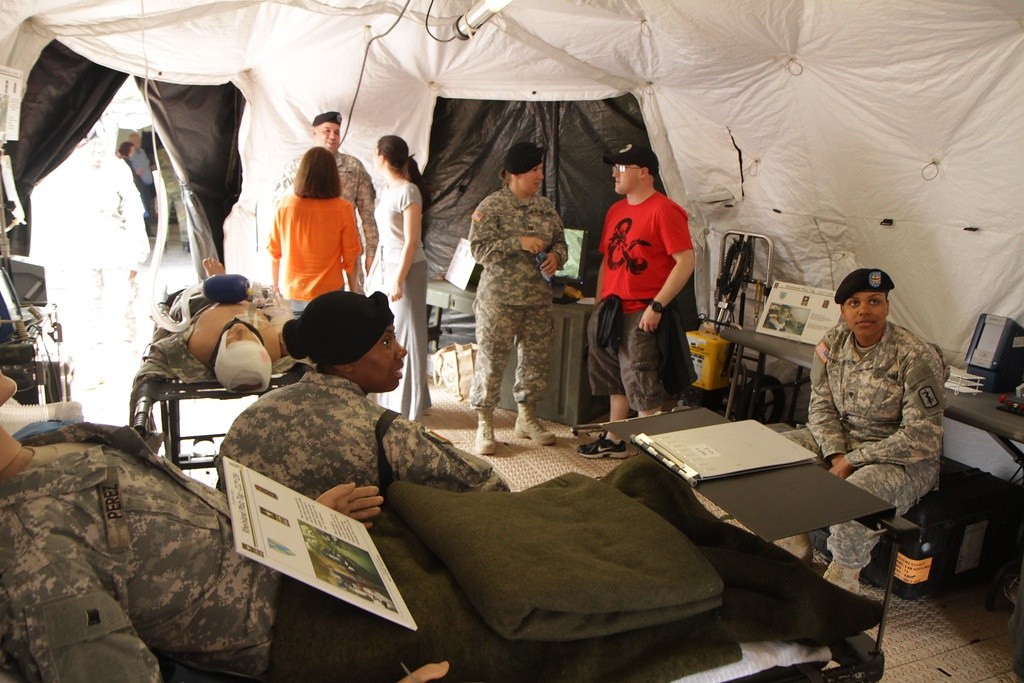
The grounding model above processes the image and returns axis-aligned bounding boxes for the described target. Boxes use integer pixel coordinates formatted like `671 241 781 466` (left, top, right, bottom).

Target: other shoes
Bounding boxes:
182 242 190 253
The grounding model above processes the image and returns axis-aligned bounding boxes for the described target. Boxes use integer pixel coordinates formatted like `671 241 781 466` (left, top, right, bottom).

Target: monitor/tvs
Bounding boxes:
550 224 592 303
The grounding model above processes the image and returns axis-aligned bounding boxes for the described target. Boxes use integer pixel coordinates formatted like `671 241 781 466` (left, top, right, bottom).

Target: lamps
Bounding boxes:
425 0 512 42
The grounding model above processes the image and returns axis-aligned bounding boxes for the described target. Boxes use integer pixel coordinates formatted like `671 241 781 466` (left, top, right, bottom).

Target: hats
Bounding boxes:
282 290 395 365
313 112 342 125
603 143 659 176
834 268 894 304
503 142 544 175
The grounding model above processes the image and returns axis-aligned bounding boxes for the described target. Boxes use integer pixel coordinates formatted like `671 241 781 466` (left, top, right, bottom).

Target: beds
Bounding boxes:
150 631 884 683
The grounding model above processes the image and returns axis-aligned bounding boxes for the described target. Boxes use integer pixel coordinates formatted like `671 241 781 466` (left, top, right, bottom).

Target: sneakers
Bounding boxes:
577 432 628 458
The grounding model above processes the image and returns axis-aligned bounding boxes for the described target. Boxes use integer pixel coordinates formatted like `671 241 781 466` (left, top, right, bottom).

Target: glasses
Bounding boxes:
611 166 641 173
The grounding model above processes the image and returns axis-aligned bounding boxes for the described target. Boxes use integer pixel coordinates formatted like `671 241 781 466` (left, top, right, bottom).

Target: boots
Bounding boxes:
475 408 495 455
514 403 555 445
822 560 861 593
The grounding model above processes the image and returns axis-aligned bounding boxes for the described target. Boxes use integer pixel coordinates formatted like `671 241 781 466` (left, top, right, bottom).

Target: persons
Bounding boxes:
374 135 432 422
266 147 360 318
169 257 291 393
576 144 695 458
0 371 449 683
271 111 379 297
468 141 569 454
215 291 511 502
773 268 951 593
116 133 157 240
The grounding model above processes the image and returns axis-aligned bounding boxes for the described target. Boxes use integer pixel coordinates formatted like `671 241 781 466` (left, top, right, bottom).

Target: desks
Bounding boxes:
426 281 596 426
602 408 924 655
719 328 1024 487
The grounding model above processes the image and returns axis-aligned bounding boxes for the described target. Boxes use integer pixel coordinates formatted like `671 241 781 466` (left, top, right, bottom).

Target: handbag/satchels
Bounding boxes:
432 342 478 402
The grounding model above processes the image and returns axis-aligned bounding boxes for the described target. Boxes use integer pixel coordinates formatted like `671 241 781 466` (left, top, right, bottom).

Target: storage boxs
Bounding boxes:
555 228 589 279
808 504 989 600
2 260 48 307
445 238 484 290
686 330 729 390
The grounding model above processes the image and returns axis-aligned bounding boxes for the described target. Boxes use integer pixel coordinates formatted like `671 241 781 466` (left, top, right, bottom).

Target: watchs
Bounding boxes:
649 300 665 313
